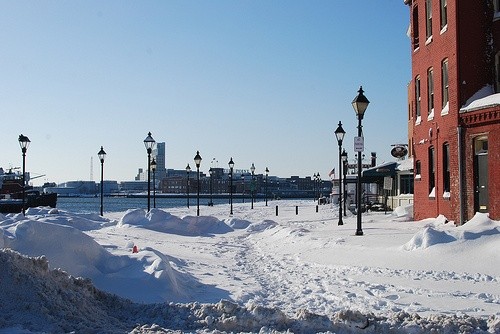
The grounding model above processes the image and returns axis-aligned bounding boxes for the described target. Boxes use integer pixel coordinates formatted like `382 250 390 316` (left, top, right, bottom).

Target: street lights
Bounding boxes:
270 180 273 201
350 85 371 236
264 167 269 206
341 149 349 216
313 172 325 202
151 158 158 208
208 167 214 206
97 145 107 217
252 177 256 202
143 131 156 213
227 157 235 214
250 163 256 209
194 150 203 217
277 181 280 200
18 134 32 216
228 172 232 204
185 163 191 208
263 178 266 201
333 121 347 227
241 173 245 203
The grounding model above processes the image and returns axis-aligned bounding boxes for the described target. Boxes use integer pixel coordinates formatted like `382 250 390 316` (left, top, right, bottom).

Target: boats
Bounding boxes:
0 166 58 214
